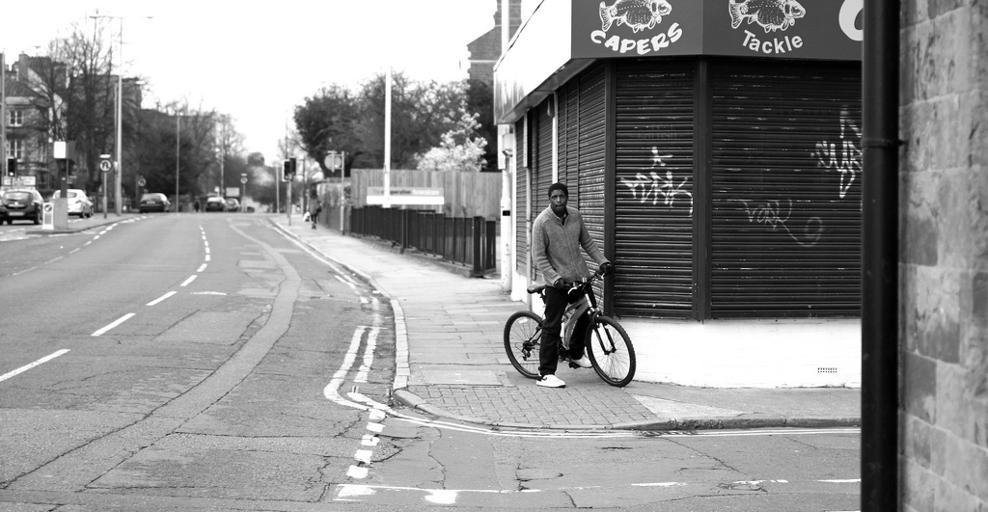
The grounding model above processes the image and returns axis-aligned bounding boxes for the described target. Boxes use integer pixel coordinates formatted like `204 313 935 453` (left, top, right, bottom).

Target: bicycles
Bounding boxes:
503 262 636 388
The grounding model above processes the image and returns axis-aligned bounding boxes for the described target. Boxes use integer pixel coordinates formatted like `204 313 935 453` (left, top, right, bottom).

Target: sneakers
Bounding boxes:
311 225 317 229
571 353 593 369
536 373 567 388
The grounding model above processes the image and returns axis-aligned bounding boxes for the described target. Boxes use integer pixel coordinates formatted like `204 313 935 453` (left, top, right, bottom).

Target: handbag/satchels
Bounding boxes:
316 206 322 212
303 211 311 221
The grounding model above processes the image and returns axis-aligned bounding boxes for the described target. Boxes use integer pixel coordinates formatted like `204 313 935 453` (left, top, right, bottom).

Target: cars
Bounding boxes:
0 189 45 224
206 197 240 211
52 189 95 218
140 193 171 213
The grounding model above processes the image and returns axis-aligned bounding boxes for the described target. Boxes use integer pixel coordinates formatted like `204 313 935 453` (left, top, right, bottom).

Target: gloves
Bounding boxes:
554 278 571 291
599 262 615 278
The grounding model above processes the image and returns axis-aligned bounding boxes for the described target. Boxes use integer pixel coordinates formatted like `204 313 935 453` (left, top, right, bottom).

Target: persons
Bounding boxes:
308 189 321 229
195 195 200 211
531 183 613 387
